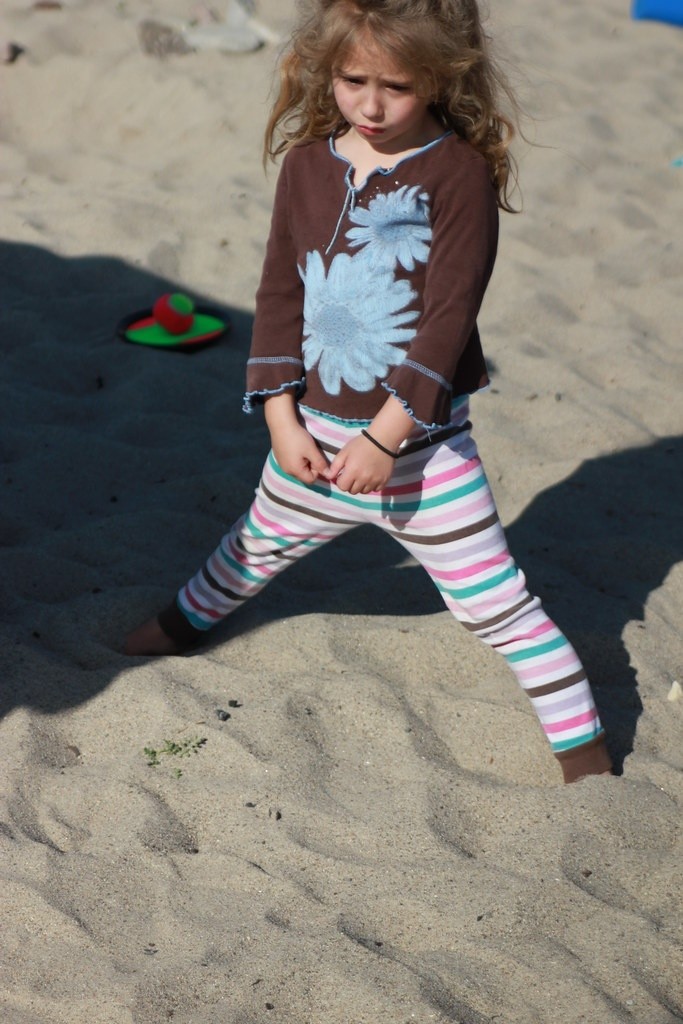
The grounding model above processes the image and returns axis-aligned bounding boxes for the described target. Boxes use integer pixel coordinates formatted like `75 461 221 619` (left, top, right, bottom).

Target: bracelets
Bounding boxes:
360 427 403 459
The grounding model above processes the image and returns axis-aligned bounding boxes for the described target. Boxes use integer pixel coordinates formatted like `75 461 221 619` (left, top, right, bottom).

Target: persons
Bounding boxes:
113 0 631 782
